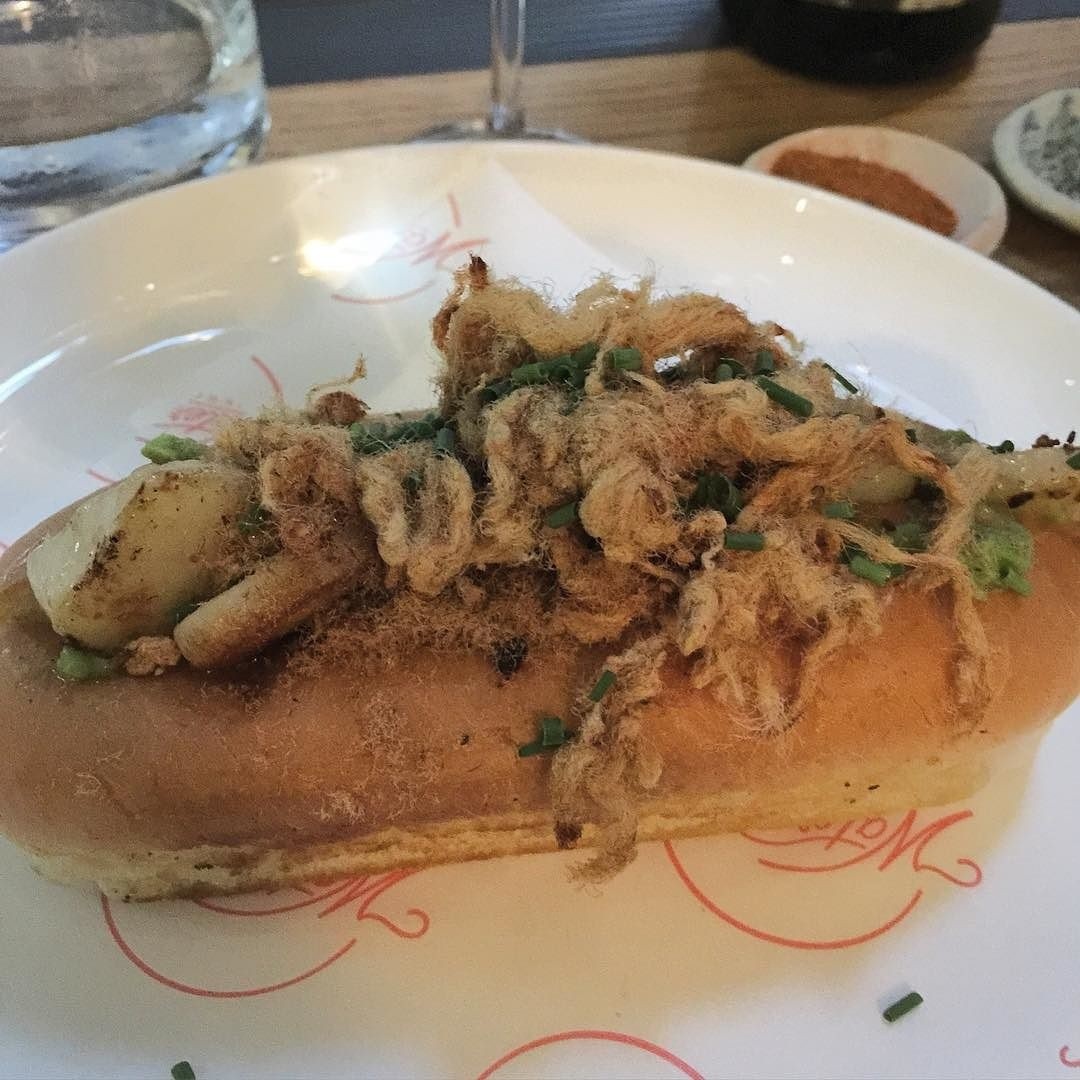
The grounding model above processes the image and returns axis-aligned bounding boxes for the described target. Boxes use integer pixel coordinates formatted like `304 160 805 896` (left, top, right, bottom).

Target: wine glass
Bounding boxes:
404 0 589 143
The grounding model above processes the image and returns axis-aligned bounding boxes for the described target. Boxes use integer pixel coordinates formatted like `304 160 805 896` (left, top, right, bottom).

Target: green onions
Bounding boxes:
171 1062 196 1080
59 346 1079 754
882 993 927 1022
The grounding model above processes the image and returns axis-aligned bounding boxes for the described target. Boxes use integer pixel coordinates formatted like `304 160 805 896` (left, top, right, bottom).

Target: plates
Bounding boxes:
1 142 1080 1080
737 122 1007 259
990 86 1080 230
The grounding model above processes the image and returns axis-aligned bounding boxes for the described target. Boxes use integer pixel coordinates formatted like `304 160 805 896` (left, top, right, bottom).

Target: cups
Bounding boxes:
1 0 266 250
